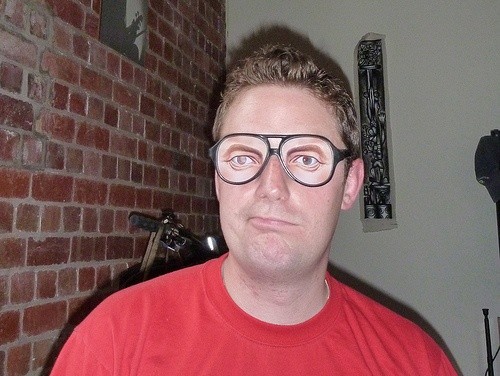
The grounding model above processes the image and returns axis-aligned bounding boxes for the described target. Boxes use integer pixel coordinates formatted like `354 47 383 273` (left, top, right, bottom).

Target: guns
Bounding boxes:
127 208 221 282
481 306 499 376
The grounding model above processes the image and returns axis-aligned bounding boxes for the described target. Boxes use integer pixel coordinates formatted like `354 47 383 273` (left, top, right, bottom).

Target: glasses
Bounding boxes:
208 131 351 188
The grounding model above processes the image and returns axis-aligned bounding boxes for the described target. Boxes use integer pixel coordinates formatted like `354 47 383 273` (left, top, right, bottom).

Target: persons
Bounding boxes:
44 43 460 376
